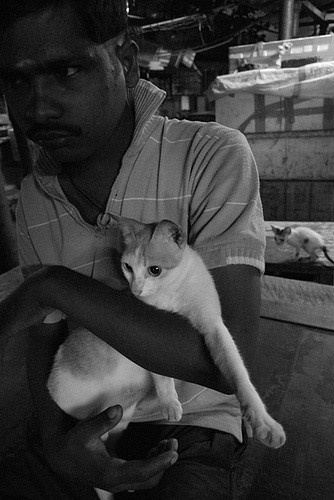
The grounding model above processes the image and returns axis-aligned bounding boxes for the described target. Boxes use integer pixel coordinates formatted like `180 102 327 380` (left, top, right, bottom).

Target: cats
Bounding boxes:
47 212 287 500
271 224 334 265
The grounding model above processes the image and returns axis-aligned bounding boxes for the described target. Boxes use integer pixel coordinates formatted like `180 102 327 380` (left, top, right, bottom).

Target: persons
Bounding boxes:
0 0 266 500
